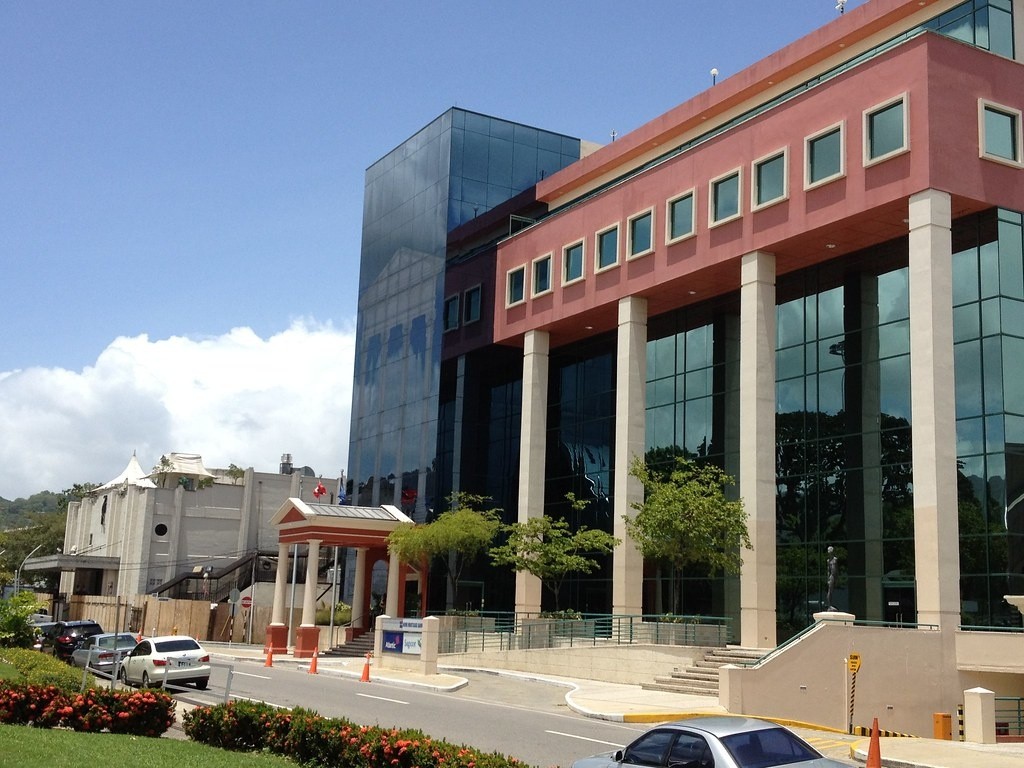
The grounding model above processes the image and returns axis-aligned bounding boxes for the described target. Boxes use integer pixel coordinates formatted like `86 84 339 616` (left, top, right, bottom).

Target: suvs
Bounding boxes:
39 618 107 665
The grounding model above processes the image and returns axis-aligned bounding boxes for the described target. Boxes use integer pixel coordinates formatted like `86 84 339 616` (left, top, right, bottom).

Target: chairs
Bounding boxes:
734 743 756 763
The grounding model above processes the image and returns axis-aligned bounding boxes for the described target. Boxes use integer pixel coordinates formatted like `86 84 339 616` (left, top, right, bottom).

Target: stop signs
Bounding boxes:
240 596 252 609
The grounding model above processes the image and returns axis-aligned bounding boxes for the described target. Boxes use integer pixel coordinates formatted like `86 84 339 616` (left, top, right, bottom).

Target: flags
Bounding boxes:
338 478 345 500
313 482 326 497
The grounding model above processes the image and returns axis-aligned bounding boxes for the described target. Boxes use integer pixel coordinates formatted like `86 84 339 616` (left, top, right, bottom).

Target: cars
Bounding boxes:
23 621 57 652
119 635 212 692
570 717 864 768
70 632 138 680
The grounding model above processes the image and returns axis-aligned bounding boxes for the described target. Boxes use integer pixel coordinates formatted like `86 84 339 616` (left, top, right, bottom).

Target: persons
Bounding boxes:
826 547 839 611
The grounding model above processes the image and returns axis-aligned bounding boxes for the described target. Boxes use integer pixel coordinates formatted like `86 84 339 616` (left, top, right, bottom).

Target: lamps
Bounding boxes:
609 127 617 142
710 66 720 85
834 0 848 16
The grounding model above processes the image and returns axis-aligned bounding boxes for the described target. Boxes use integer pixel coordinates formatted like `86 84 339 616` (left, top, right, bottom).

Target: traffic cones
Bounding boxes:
136 627 143 643
307 646 319 675
859 718 884 768
357 650 373 683
263 642 275 668
